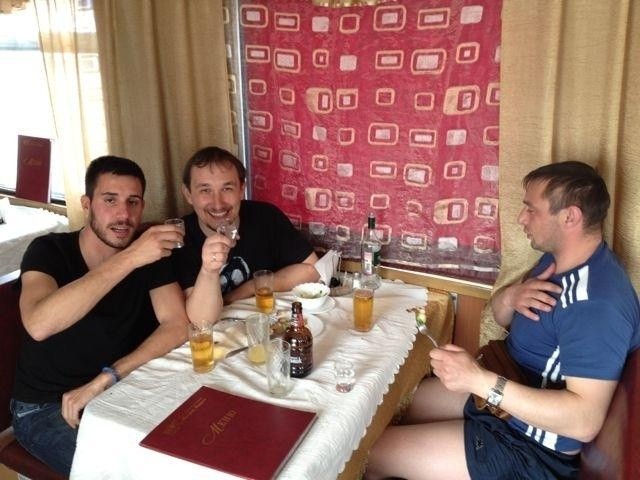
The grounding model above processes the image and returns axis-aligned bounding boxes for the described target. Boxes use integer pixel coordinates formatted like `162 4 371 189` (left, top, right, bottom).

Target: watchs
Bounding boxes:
485 374 507 409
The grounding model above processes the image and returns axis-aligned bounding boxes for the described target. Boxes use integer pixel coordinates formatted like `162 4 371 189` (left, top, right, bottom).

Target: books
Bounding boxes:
140 386 317 480
16 132 50 202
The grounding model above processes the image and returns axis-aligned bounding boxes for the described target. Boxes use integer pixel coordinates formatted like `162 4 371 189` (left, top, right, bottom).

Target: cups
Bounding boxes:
165 219 185 248
265 340 291 399
187 319 215 375
253 269 275 312
247 312 270 364
352 271 375 332
334 358 356 393
216 216 240 248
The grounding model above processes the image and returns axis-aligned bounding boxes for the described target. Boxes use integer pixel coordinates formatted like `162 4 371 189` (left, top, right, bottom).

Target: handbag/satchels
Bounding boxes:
472 268 533 422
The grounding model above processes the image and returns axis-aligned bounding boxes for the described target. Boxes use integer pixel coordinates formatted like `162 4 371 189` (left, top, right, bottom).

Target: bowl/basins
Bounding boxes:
293 282 330 309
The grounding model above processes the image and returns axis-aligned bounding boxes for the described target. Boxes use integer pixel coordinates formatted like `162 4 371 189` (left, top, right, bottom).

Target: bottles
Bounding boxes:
361 212 382 289
281 302 314 377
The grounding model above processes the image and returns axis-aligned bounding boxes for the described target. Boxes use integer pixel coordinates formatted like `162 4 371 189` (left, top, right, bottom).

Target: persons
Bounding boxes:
171 147 320 330
365 160 640 480
6 155 193 476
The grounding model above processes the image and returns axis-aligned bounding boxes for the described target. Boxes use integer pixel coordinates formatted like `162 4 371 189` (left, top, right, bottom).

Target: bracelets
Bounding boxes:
101 365 118 384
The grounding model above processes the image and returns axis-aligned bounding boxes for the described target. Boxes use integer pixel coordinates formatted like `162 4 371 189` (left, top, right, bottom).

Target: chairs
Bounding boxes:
0 267 74 480
576 341 640 479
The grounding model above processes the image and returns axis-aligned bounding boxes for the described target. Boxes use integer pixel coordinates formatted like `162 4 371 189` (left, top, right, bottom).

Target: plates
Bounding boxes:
312 297 336 313
272 312 324 342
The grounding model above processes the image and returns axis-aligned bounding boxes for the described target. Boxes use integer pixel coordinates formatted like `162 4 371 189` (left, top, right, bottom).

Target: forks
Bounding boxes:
415 318 438 348
